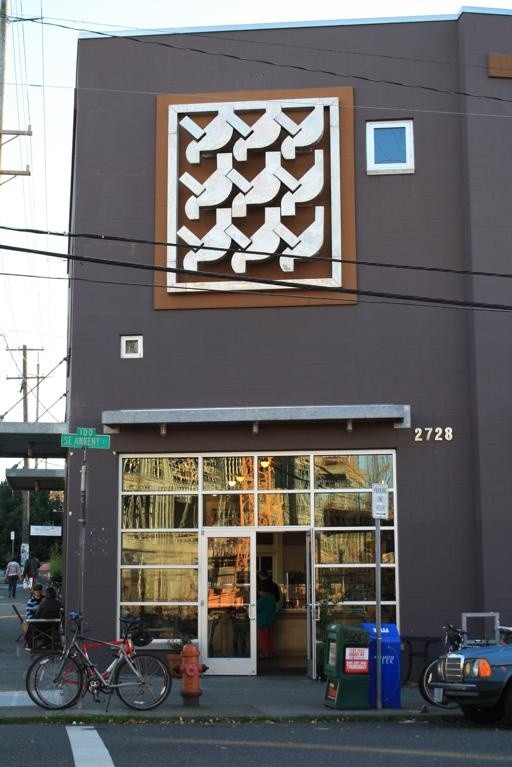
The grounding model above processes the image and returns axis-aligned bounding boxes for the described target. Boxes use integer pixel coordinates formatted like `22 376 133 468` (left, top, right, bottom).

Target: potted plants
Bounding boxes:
166 633 193 679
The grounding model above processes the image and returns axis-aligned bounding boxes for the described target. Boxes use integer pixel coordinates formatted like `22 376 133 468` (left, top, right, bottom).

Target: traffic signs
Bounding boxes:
371 482 389 519
60 433 111 450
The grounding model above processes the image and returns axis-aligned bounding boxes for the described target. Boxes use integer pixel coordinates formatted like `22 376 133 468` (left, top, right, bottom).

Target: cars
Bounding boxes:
430 643 512 724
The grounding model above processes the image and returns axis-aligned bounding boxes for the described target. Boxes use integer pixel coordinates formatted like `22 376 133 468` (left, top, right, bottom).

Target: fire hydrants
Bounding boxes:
173 641 209 706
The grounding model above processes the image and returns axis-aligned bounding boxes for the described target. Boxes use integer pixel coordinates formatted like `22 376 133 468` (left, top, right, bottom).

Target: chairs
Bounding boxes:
10 602 62 650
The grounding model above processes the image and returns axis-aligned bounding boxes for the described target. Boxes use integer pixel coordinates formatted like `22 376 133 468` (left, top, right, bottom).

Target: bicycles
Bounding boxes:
26 610 173 714
419 621 512 708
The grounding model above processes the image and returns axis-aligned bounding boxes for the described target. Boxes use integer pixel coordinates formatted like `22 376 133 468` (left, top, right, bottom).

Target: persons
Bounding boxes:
256 582 277 660
257 571 281 603
22 584 46 637
21 551 40 589
24 588 61 650
5 557 21 598
